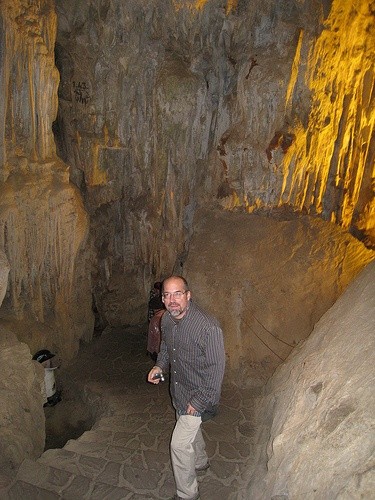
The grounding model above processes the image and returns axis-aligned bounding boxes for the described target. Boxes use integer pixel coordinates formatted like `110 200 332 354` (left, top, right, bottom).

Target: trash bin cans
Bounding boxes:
32 349 61 407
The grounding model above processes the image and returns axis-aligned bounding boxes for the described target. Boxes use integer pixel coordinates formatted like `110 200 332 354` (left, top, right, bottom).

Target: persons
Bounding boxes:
147 282 167 365
148 275 225 500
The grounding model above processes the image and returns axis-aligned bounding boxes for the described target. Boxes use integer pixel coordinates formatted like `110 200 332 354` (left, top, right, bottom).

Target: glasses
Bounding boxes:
162 291 187 299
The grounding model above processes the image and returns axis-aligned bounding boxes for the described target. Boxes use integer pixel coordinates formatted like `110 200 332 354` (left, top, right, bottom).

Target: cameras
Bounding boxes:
153 373 164 382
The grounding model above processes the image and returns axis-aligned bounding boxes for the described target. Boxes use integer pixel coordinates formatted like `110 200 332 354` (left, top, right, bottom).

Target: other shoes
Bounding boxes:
199 463 210 470
173 491 200 500
